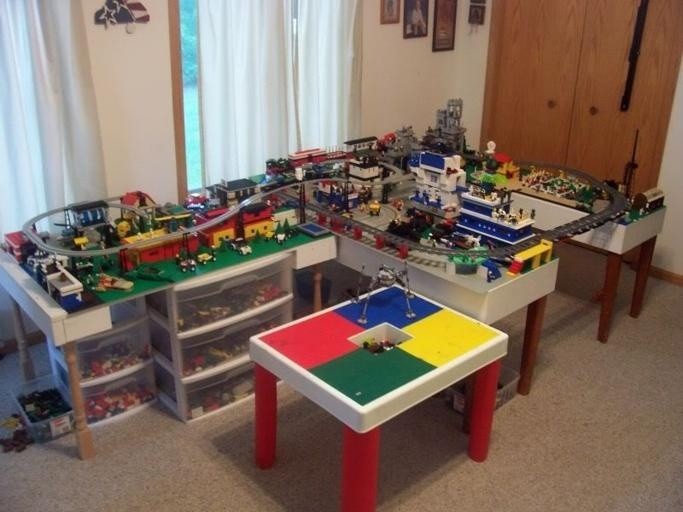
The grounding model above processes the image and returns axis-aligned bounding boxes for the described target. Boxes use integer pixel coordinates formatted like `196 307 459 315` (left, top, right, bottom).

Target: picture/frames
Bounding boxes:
403 0 428 39
468 4 485 25
432 0 457 52
380 0 400 24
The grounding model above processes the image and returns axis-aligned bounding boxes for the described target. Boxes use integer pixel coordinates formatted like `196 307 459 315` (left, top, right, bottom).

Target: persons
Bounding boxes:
383 0 397 22
471 7 482 20
411 0 427 37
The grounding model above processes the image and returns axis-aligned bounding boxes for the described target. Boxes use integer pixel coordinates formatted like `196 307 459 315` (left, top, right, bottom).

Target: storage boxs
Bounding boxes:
9 373 74 444
443 365 522 418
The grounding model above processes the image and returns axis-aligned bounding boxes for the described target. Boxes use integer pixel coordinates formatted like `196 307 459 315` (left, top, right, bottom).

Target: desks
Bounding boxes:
249 174 560 394
249 285 509 512
461 164 665 343
0 220 338 459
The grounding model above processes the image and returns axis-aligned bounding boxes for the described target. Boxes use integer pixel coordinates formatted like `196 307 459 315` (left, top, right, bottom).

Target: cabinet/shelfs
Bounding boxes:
145 254 294 424
47 297 158 429
478 0 683 264
